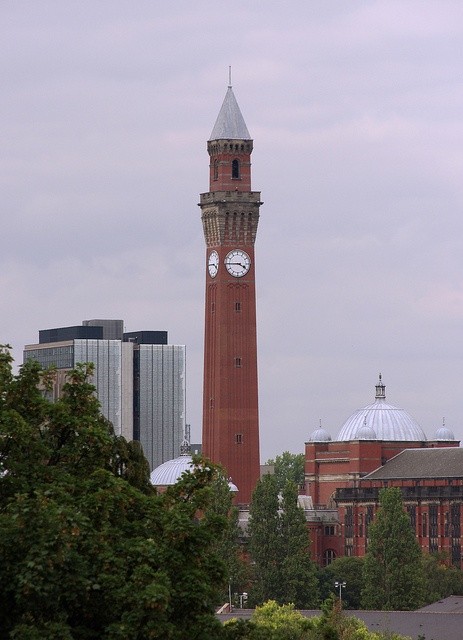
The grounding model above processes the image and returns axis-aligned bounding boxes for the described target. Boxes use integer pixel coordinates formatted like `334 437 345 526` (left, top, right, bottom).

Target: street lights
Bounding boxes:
225 578 232 611
333 581 346 610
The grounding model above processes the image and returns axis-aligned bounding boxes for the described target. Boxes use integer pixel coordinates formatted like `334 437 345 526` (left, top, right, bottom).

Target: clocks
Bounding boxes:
224 248 251 278
207 250 219 279
